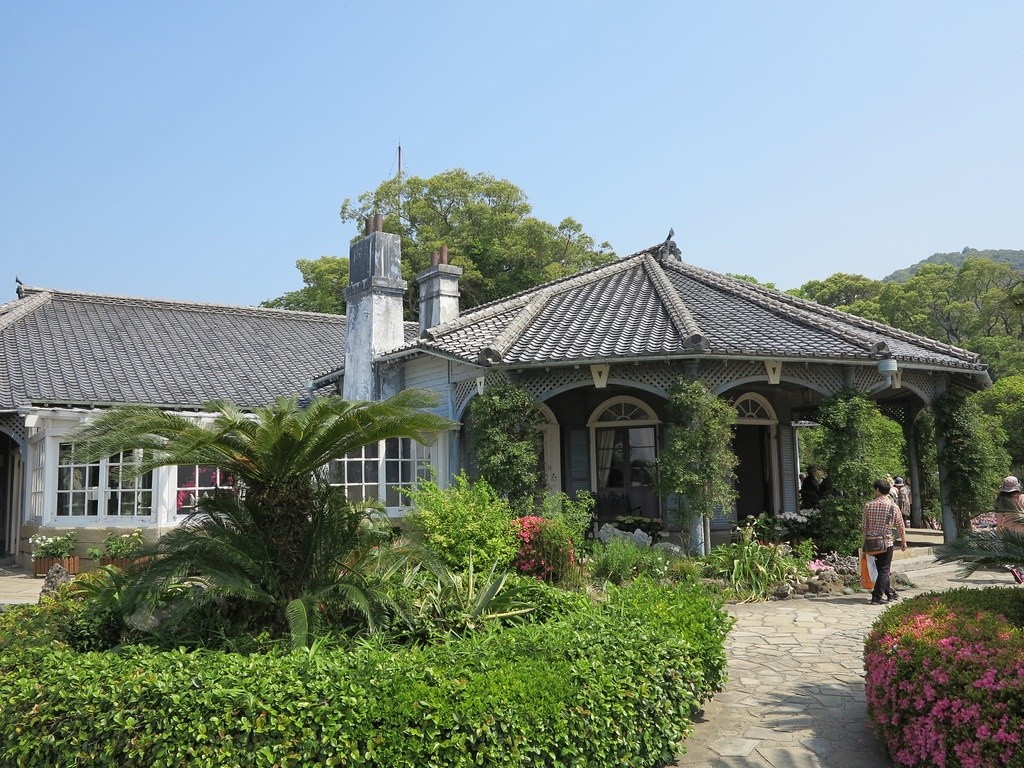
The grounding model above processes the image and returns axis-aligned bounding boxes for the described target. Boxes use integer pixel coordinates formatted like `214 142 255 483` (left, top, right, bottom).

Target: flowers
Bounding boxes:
27 531 76 562
86 529 144 562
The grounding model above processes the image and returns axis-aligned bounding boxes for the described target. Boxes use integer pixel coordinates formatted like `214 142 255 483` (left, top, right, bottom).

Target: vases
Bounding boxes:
99 557 148 572
34 556 79 578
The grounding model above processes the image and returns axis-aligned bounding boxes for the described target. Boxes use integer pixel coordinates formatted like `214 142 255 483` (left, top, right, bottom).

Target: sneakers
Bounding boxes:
1011 569 1024 584
888 594 899 602
871 598 889 604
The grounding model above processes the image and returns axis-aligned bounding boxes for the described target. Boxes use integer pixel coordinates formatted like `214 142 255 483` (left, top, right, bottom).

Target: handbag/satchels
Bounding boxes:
858 548 878 589
862 537 887 554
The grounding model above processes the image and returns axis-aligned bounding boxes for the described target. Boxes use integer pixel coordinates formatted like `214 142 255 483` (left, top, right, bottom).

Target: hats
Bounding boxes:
893 478 904 486
1000 477 1021 493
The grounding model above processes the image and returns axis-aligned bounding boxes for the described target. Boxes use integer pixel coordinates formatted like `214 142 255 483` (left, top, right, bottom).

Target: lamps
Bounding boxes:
800 387 814 403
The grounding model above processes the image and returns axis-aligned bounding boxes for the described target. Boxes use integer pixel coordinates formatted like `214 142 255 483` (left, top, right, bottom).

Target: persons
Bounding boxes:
799 466 842 508
886 474 912 528
861 480 907 605
995 476 1024 584
